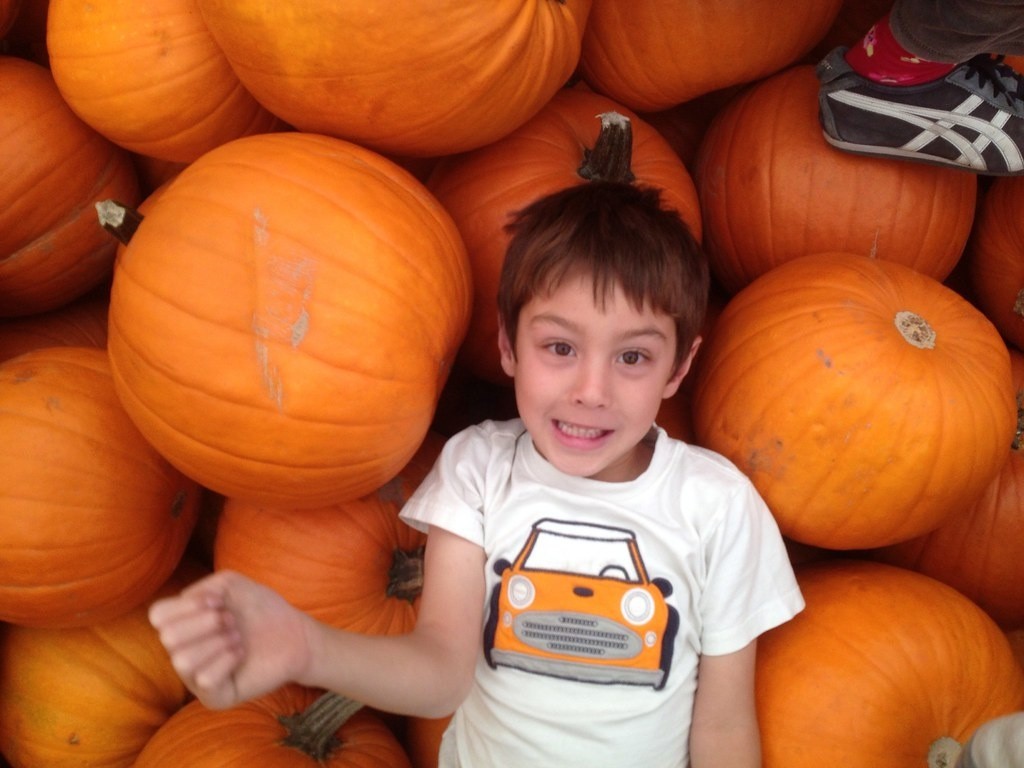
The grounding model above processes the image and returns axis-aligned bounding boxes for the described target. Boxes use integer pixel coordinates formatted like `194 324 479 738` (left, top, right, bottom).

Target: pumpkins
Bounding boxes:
0 0 1024 768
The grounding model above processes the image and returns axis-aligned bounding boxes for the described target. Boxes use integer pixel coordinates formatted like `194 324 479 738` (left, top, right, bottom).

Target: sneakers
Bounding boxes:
816 45 1024 176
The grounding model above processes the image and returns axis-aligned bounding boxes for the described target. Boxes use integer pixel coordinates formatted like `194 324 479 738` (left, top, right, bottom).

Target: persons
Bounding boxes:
148 178 807 768
815 0 1024 176
